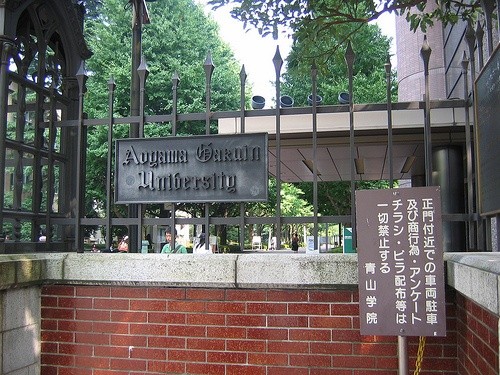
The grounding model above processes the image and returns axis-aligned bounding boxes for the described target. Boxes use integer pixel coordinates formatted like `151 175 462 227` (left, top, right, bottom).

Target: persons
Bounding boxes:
291 233 299 253
145 234 155 249
109 243 115 251
193 233 213 254
161 226 187 253
90 244 99 253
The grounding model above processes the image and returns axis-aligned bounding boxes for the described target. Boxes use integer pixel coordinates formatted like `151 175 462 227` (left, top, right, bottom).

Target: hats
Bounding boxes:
199 233 205 238
